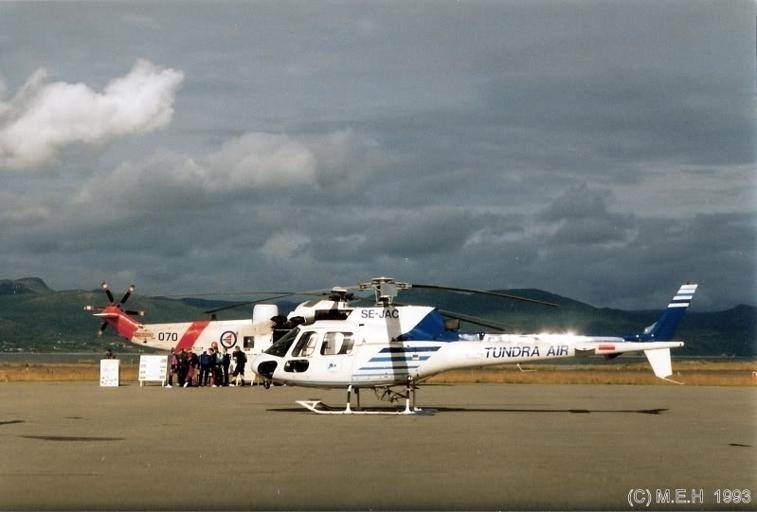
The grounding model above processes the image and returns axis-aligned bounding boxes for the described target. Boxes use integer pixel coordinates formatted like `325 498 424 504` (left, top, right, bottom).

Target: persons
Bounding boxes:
228 345 247 387
163 345 231 388
